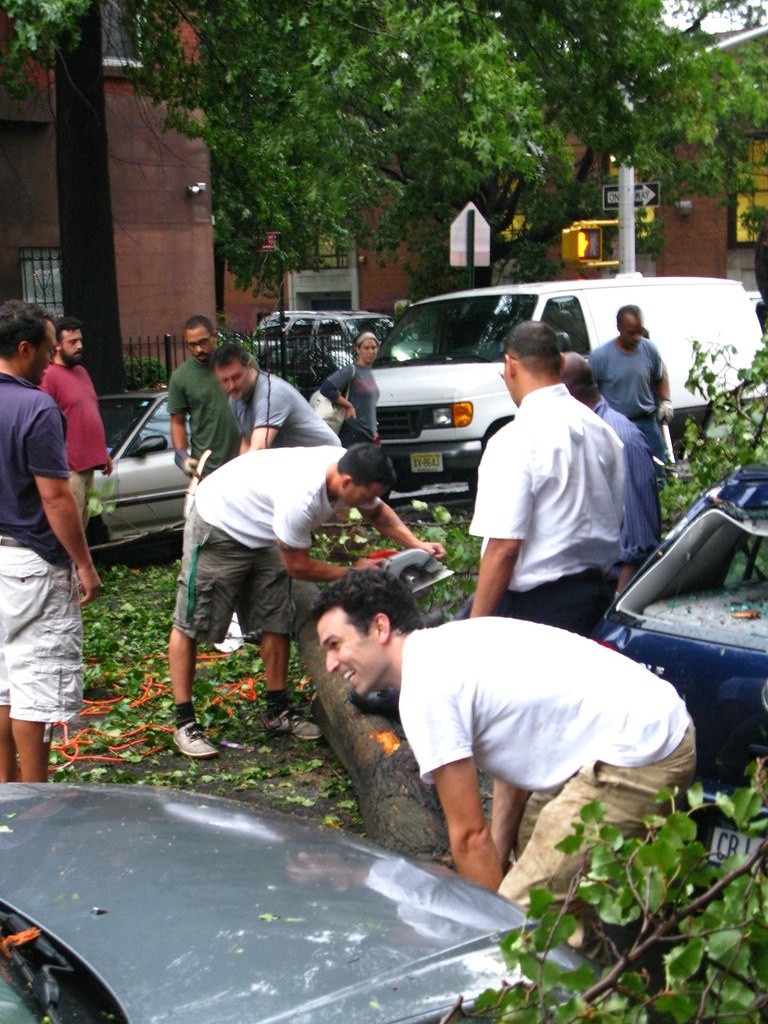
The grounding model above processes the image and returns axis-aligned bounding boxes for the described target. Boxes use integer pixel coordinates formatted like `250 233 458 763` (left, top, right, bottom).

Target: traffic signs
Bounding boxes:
601 182 661 209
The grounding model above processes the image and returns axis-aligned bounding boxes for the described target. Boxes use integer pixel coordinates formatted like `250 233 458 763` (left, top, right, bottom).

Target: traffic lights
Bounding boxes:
562 228 603 261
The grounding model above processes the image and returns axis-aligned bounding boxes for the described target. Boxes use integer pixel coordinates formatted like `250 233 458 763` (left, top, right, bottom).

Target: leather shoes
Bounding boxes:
348 689 401 725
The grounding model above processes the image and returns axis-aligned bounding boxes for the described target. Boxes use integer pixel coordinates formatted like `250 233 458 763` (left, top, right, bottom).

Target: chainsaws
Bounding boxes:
369 548 455 597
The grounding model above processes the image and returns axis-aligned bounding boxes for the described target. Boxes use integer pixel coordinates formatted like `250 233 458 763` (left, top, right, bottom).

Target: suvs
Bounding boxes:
249 309 397 367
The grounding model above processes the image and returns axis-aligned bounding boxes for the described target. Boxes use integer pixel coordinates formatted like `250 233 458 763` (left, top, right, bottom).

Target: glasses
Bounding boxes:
498 357 517 380
187 334 213 347
622 327 645 336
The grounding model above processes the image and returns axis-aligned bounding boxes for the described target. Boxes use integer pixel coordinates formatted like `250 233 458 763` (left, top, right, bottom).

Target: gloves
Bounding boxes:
657 399 674 424
175 449 199 477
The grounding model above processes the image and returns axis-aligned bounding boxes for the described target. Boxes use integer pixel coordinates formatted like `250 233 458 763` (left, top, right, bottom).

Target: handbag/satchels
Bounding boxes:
310 364 355 436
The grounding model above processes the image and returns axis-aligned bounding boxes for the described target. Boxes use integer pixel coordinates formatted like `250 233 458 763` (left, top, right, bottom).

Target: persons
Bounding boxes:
460 300 673 632
322 331 380 446
41 317 112 535
167 315 259 481
211 344 341 446
173 439 446 760
312 571 698 918
0 300 102 785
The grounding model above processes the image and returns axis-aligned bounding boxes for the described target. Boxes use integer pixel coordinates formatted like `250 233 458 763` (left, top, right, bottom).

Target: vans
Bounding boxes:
334 275 768 500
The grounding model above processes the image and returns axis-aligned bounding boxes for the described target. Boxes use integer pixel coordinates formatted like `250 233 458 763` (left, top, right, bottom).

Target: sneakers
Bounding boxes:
261 709 323 738
173 721 219 757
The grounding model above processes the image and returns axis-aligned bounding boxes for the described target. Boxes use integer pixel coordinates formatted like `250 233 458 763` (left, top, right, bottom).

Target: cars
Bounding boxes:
83 391 196 549
582 466 767 883
745 290 764 310
1 784 605 1022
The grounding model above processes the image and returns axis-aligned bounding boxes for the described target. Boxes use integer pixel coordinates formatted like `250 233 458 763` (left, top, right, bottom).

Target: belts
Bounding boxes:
0 538 29 548
